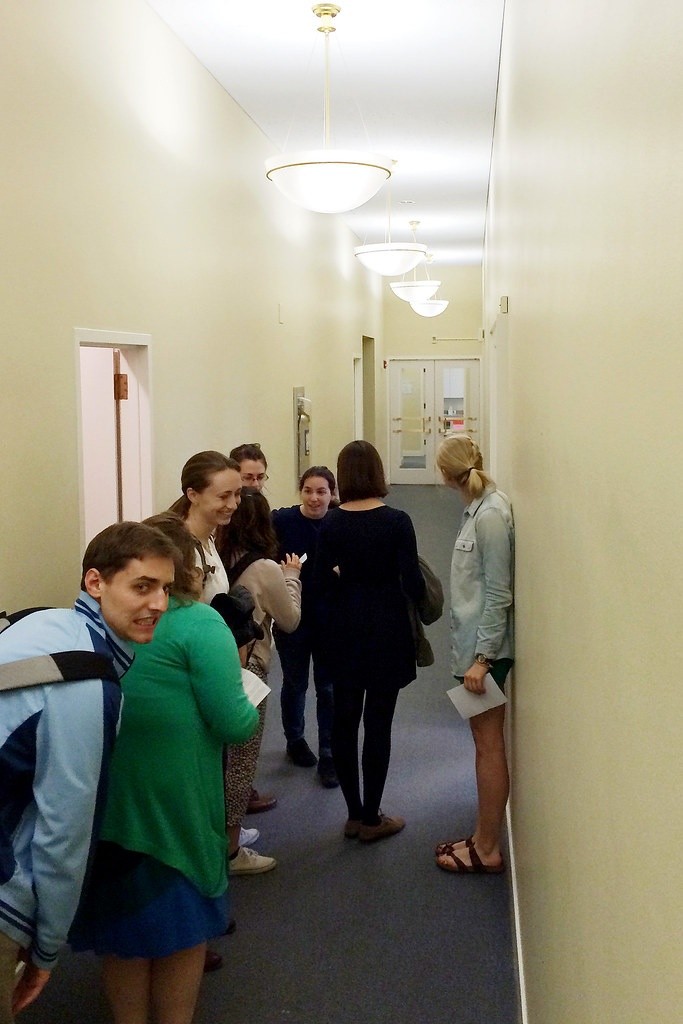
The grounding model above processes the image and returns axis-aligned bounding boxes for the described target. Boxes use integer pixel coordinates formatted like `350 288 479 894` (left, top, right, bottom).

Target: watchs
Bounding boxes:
475 653 493 666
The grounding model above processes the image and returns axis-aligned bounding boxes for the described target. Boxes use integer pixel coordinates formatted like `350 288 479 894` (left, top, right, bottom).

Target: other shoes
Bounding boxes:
222 919 237 935
287 738 317 766
318 758 338 788
204 952 222 971
360 816 406 843
246 790 277 813
345 821 360 839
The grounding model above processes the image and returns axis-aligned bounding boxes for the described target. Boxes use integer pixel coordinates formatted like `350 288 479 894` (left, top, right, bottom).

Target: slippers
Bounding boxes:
436 836 504 874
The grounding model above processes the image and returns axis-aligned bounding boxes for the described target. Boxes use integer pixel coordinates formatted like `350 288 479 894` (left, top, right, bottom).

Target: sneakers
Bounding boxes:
238 826 260 847
227 847 276 875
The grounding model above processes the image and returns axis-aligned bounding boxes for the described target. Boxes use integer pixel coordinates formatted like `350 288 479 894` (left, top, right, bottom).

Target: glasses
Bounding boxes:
241 473 269 482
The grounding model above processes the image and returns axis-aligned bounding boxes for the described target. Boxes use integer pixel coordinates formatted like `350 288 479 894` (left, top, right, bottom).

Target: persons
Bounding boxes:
0 441 339 1024
311 440 424 843
436 435 514 875
